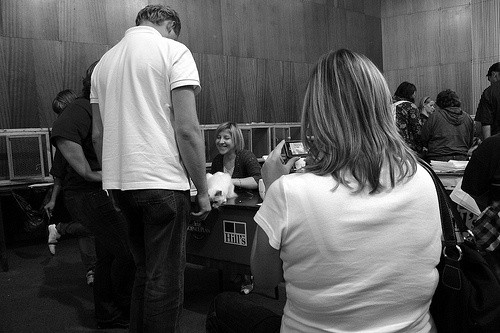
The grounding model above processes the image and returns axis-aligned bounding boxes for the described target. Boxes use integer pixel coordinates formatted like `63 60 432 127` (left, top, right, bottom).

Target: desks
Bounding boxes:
186 188 285 299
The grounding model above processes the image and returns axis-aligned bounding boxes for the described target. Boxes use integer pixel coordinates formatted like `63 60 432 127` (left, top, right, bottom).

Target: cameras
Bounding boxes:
283 138 311 159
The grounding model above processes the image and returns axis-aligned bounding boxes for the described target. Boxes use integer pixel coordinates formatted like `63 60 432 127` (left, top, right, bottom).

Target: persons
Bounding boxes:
90 5 211 333
392 61 500 256
206 49 444 333
206 121 263 188
44 88 96 286
52 60 138 328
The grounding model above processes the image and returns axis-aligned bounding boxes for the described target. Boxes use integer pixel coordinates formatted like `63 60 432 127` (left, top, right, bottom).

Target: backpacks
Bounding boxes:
392 100 410 123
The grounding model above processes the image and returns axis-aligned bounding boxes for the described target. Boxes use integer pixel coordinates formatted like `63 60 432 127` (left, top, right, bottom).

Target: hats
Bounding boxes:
436 89 461 108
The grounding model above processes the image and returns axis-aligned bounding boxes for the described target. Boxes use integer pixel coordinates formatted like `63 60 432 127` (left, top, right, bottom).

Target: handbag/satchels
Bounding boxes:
429 239 500 333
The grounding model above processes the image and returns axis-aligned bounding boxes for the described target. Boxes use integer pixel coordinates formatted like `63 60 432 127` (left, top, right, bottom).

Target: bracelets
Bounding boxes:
239 179 241 187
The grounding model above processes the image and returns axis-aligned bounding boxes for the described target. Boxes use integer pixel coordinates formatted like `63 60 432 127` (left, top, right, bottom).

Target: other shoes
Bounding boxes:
96 318 130 328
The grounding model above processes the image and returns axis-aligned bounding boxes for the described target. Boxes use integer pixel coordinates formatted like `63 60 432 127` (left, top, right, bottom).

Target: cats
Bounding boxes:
191 172 238 211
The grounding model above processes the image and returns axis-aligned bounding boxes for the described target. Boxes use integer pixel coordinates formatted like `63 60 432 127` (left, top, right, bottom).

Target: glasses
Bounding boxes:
413 94 416 98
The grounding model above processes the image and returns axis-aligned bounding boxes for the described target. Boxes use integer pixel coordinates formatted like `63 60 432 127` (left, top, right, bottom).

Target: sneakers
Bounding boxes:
46 224 61 256
85 270 94 285
